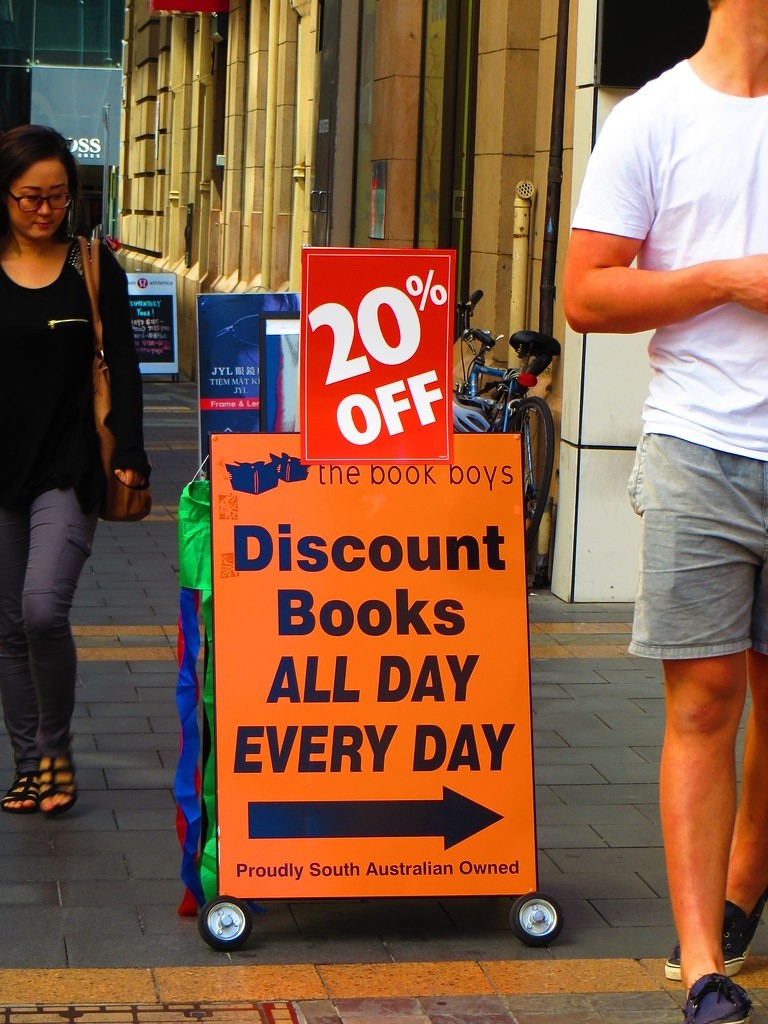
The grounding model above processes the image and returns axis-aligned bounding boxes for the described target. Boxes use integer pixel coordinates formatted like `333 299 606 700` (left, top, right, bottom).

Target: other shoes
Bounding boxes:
663 882 764 981
682 973 753 1021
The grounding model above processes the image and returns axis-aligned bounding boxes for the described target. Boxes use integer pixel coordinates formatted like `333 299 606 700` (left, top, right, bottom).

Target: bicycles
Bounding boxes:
455 289 564 553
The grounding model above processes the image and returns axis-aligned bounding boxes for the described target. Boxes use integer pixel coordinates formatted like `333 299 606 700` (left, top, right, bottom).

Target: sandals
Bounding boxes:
38 753 80 817
2 766 40 816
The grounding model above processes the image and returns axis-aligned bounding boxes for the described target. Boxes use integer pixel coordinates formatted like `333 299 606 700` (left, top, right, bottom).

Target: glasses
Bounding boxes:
6 187 73 213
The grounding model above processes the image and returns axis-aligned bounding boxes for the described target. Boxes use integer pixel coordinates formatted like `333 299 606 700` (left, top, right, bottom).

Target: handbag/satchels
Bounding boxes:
90 317 154 523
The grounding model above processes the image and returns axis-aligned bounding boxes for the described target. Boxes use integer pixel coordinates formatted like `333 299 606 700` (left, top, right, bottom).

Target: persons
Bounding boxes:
0 125 148 816
563 0 768 1024
